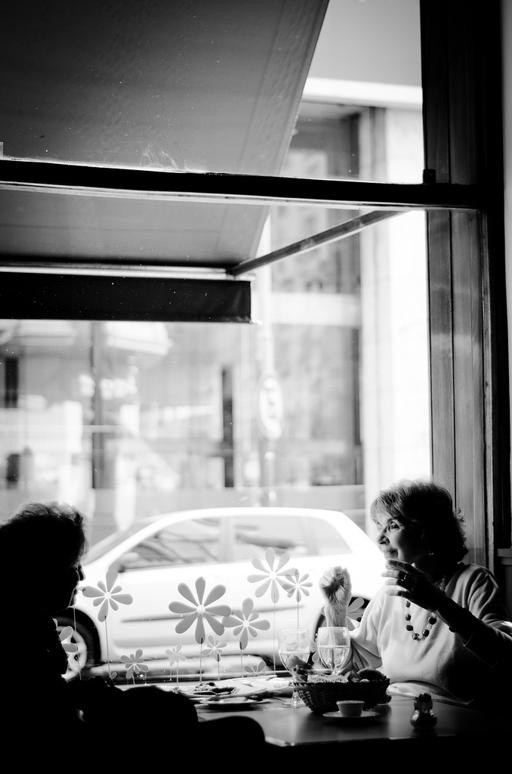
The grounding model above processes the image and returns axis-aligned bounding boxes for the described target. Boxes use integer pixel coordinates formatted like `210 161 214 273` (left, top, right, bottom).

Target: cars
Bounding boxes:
58 505 392 675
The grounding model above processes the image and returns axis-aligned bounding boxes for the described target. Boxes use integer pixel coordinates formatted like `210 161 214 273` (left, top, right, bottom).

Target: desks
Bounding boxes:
113 676 466 774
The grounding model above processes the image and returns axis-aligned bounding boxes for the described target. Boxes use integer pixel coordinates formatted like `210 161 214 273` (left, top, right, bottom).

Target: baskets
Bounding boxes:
293 677 392 715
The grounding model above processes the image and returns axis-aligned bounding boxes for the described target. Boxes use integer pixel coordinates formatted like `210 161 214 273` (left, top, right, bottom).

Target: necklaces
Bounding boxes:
404 575 449 640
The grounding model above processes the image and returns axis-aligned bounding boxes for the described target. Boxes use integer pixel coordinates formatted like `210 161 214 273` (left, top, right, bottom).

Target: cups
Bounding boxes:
336 700 365 716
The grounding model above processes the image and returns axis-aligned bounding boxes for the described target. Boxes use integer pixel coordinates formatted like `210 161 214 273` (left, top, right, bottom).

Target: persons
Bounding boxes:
0 493 92 773
319 476 511 716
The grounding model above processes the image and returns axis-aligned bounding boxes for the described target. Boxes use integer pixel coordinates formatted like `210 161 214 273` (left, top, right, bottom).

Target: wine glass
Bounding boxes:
276 625 311 709
315 626 351 675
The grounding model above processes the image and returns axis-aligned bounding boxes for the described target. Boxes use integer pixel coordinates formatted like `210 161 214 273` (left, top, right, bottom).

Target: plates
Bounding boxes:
322 710 381 721
198 694 274 712
